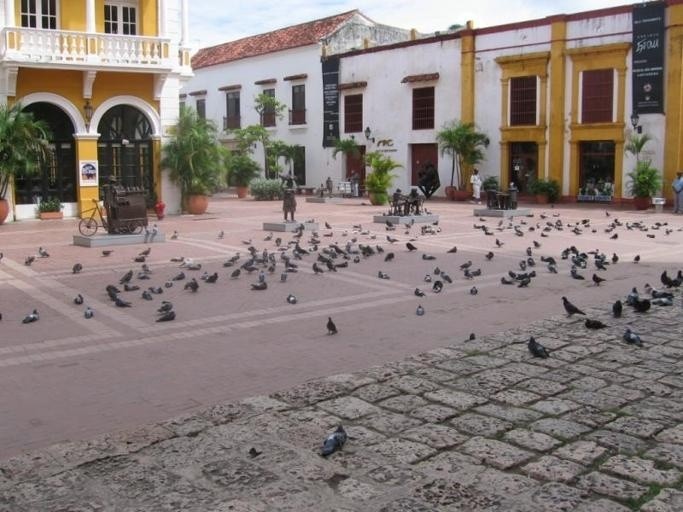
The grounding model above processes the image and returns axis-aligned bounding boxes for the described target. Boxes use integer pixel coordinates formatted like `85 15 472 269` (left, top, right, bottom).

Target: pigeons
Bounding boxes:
172 230 179 239
224 210 683 334
219 231 224 239
528 334 552 363
465 331 475 343
22 308 39 323
318 422 349 454
72 246 220 322
24 255 35 265
38 246 50 258
623 328 642 346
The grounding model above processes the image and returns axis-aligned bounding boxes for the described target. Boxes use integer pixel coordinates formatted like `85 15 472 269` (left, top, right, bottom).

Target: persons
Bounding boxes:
583 176 613 199
672 171 683 215
351 170 363 197
470 167 482 204
326 177 333 193
100 174 121 234
417 159 434 186
281 169 298 222
407 187 421 215
393 188 409 216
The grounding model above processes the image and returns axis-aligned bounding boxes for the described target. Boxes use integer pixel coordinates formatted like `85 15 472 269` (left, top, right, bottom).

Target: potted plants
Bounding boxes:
527 177 560 204
361 149 404 206
435 118 490 201
155 106 261 215
622 132 662 210
38 198 63 219
0 101 54 225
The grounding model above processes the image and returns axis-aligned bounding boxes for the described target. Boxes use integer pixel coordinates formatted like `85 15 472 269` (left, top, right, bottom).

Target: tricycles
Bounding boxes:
77 184 148 240
